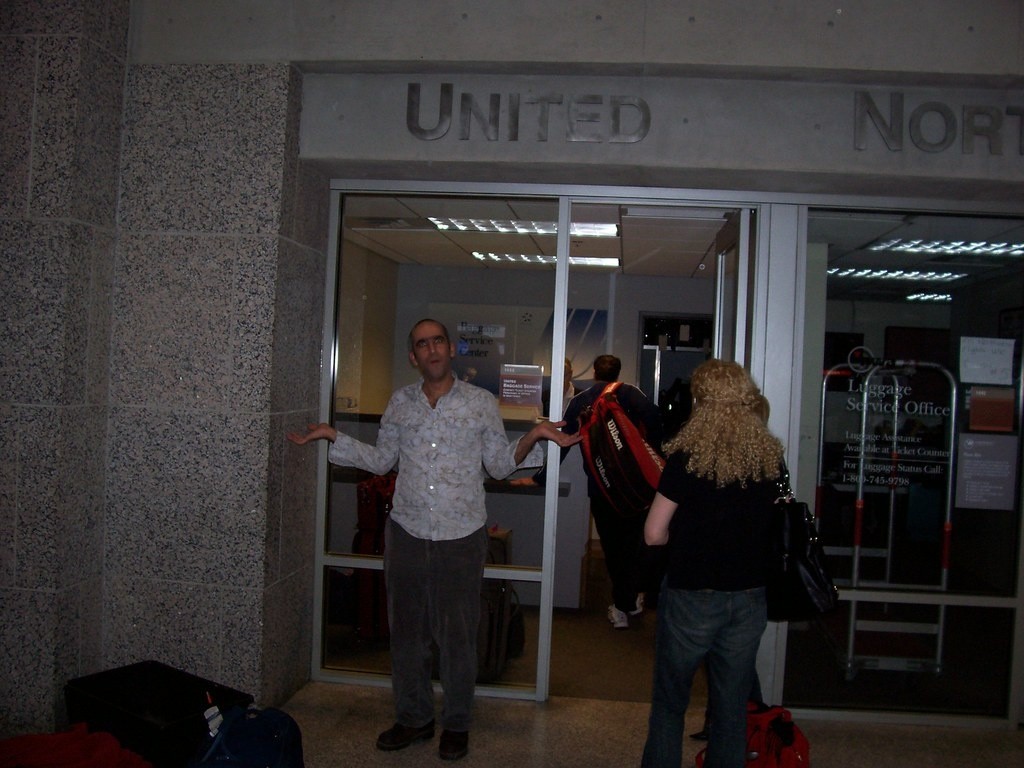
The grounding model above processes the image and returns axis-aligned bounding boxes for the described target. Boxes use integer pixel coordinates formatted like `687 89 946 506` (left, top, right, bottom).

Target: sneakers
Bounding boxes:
439 728 469 759
375 717 436 750
606 603 629 629
629 592 647 615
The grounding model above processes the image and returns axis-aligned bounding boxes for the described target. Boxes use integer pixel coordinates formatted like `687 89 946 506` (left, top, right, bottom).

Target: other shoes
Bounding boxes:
689 730 707 741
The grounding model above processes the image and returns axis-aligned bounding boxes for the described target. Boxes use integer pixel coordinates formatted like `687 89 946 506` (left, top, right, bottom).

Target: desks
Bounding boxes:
331 466 570 498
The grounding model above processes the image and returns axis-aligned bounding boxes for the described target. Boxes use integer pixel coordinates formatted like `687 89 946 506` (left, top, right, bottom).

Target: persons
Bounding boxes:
284 318 585 762
460 364 491 392
541 352 793 768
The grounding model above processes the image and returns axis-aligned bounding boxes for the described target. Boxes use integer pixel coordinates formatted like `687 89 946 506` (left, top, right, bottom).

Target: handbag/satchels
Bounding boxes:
764 453 841 623
576 381 668 514
695 701 810 768
196 707 305 768
